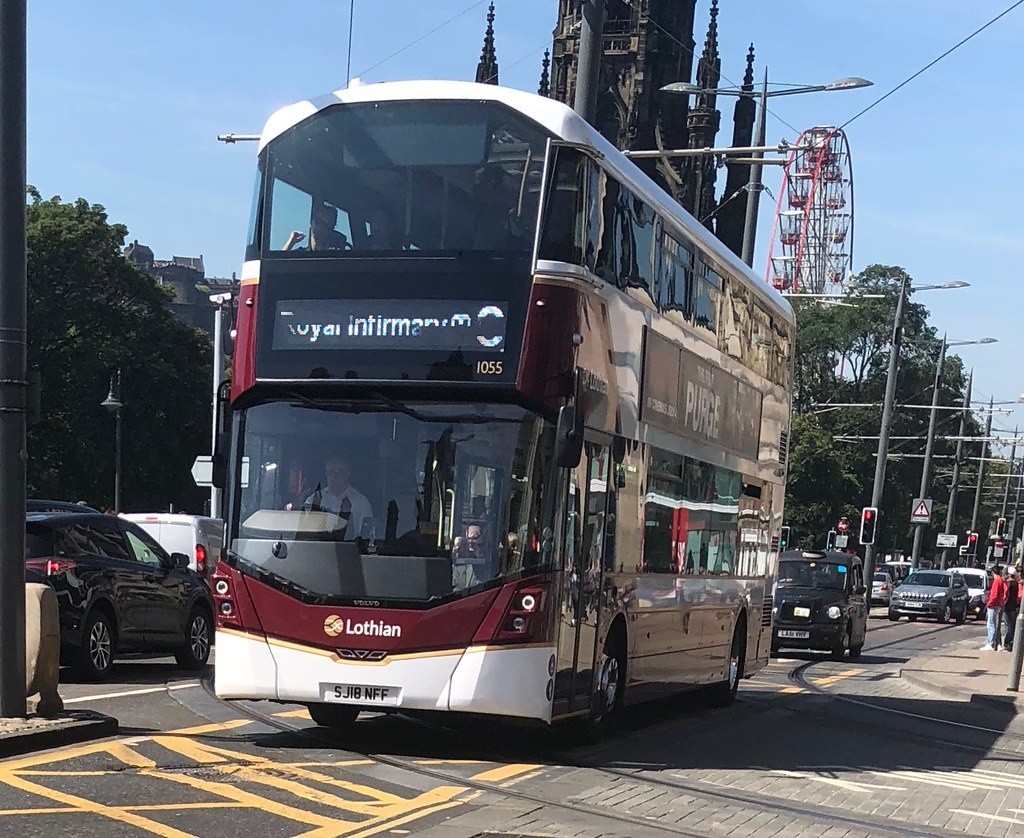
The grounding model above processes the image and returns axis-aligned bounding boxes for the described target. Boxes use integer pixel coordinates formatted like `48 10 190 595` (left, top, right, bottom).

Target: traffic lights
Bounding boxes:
967 532 979 556
997 518 1007 537
779 526 790 547
859 507 878 545
827 531 837 549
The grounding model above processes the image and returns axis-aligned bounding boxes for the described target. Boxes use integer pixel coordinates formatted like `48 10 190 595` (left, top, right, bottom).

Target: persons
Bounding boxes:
452 537 478 591
103 504 113 514
350 206 392 252
281 206 338 252
980 566 1024 652
785 565 794 582
283 457 375 546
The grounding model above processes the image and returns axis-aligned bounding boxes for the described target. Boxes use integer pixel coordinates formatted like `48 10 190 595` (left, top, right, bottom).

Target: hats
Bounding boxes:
1008 567 1016 574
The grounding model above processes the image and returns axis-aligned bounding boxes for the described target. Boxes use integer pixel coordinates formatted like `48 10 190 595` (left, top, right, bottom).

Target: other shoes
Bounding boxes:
980 644 994 651
998 644 1002 651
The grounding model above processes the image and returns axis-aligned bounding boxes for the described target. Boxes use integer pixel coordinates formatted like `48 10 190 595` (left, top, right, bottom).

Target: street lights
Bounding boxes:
658 77 874 269
901 332 998 571
847 272 971 614
100 367 124 513
952 394 1024 568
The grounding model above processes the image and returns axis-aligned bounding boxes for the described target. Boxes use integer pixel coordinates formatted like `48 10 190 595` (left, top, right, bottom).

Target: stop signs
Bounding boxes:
838 521 850 532
995 539 1004 548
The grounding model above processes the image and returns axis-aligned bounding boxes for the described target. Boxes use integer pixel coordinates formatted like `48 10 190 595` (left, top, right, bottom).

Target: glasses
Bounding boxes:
313 217 334 229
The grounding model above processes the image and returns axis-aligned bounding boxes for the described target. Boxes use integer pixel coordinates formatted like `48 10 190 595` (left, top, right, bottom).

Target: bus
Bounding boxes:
210 77 796 746
882 562 922 584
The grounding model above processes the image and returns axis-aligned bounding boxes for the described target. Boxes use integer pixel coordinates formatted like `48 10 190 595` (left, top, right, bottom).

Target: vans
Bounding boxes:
117 511 223 581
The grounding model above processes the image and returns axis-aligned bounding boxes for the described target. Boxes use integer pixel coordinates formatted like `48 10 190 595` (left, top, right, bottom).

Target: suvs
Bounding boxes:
770 549 867 658
888 568 972 624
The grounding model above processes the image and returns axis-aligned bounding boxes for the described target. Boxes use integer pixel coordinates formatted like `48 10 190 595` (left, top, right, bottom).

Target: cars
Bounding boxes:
26 498 103 514
871 572 893 606
25 512 215 679
941 567 989 619
